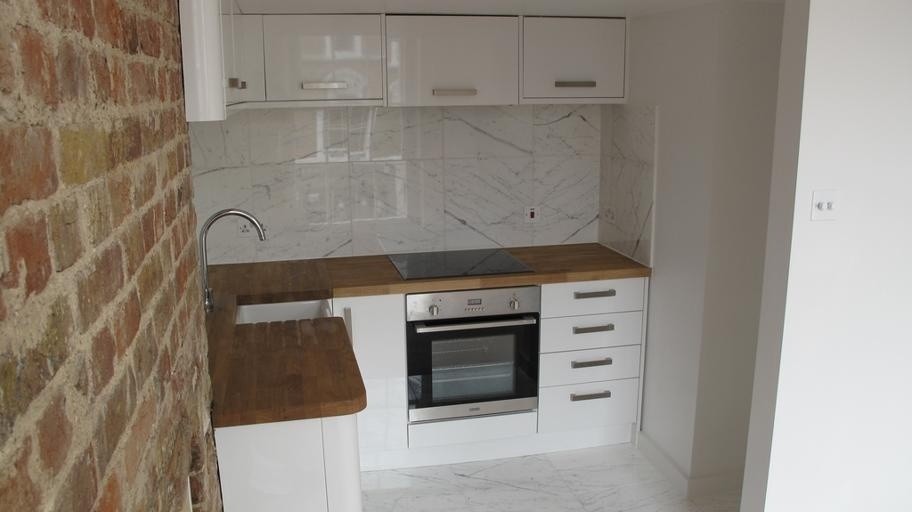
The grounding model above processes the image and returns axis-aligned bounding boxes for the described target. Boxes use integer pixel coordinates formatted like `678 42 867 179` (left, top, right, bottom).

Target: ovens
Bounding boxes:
403 285 541 423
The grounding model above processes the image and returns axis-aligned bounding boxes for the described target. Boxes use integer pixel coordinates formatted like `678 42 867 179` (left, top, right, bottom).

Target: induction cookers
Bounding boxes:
386 249 537 283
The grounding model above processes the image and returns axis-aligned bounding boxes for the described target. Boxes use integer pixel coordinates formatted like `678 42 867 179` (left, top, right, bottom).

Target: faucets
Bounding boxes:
198 208 266 314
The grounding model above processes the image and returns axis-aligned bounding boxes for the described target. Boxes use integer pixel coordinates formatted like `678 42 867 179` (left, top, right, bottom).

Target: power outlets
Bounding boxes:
239 219 270 237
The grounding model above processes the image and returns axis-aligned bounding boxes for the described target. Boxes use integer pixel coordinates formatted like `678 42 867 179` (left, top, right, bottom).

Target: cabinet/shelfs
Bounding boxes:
537 276 650 452
386 15 519 107
179 14 266 123
519 15 631 105
263 14 387 107
333 293 408 472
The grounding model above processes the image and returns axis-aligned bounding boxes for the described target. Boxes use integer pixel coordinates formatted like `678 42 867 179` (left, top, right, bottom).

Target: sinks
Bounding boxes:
231 286 344 326
